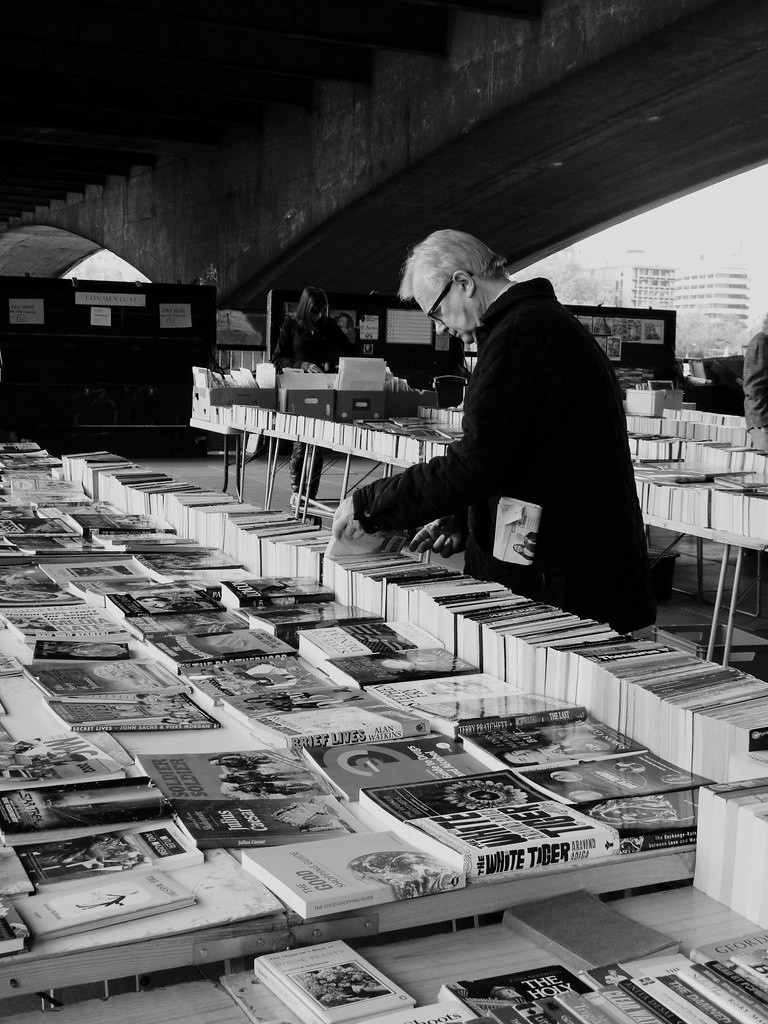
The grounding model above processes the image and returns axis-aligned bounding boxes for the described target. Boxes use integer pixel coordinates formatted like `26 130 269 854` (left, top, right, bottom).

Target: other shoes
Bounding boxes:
290 492 316 507
743 548 758 562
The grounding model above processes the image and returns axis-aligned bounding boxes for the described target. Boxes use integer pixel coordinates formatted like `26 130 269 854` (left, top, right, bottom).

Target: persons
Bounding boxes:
331 229 657 640
272 285 359 513
742 314 768 454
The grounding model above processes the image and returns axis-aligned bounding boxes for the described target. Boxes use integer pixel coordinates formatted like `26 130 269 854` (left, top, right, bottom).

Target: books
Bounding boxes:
1 356 768 1024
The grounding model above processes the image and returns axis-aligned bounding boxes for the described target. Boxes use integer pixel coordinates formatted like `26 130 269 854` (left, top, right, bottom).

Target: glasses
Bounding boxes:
428 270 473 326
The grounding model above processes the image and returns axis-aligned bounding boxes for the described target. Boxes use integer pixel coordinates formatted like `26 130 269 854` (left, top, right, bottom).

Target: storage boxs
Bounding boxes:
194 386 438 423
652 624 768 680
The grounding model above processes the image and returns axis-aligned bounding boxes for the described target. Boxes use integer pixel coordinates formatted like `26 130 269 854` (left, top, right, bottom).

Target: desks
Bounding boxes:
188 419 768 669
1 849 755 1024
